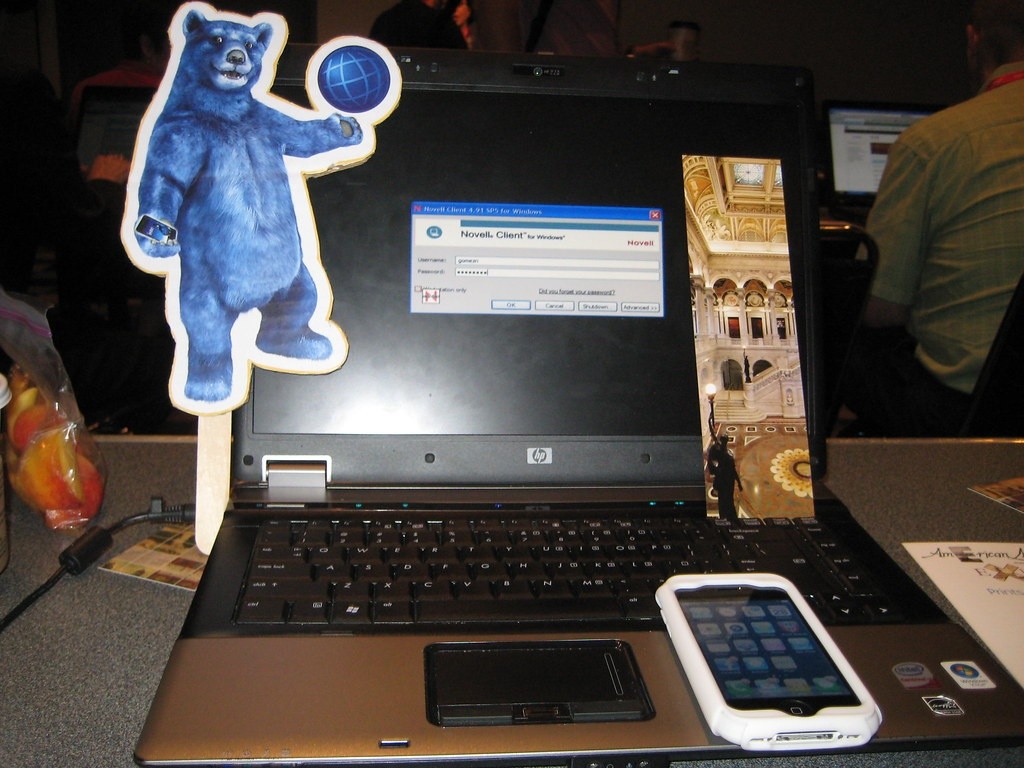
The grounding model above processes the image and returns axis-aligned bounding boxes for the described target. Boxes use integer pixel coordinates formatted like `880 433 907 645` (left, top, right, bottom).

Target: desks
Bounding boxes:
0 428 1024 768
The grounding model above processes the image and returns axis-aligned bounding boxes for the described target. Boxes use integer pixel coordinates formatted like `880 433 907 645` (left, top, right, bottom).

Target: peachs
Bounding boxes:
4 364 102 534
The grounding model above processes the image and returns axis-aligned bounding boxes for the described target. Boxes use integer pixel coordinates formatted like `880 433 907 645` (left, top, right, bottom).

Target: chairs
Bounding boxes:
816 217 881 436
851 267 1023 437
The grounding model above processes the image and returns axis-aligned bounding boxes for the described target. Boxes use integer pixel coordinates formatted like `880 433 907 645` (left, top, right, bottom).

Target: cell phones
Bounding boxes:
656 572 883 752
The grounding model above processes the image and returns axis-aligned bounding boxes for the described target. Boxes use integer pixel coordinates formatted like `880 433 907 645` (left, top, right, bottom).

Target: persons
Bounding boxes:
708 435 745 518
434 0 679 57
369 0 468 49
0 0 170 330
859 0 1024 438
426 0 472 48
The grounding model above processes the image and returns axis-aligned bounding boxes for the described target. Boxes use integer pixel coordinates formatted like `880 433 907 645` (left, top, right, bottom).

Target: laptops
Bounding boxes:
71 83 156 178
135 41 1024 768
819 95 954 228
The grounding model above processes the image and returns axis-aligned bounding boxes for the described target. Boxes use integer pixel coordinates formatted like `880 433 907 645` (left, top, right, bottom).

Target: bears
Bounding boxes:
133 9 362 403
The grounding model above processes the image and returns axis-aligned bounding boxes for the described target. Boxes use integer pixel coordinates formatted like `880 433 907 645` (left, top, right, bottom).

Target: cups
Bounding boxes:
666 19 703 62
0 374 13 577
